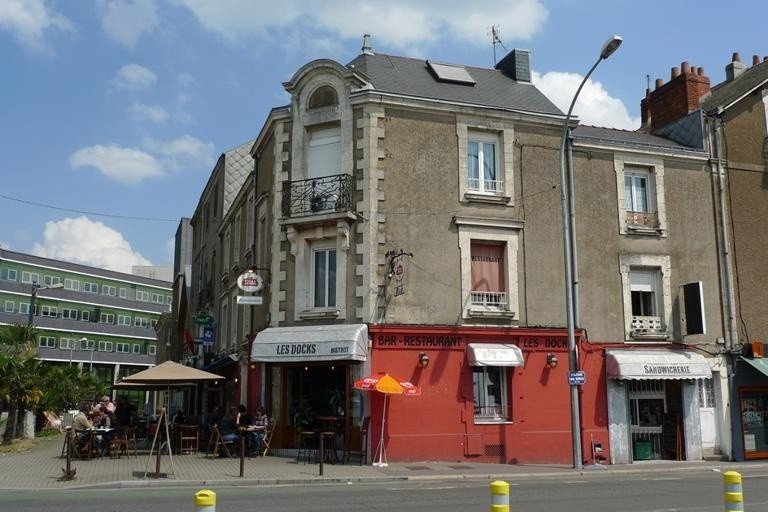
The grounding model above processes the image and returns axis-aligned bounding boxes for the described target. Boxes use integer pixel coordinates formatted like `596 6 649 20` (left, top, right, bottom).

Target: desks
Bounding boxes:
316 416 339 464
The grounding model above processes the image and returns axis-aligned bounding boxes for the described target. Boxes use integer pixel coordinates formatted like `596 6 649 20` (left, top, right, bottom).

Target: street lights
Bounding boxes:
28 281 64 330
561 36 624 468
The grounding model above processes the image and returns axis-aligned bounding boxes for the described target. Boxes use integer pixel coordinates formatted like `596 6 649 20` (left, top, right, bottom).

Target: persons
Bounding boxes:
654 406 663 425
204 404 268 457
72 395 137 455
642 407 657 426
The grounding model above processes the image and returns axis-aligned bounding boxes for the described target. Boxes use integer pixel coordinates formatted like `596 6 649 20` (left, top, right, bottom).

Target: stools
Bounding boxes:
297 432 338 465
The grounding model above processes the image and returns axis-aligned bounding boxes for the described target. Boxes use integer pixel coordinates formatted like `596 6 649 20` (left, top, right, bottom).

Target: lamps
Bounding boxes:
419 351 430 368
547 350 559 371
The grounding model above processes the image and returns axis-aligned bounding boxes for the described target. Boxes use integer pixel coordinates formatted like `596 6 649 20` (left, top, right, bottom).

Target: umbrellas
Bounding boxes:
107 359 226 455
351 372 422 467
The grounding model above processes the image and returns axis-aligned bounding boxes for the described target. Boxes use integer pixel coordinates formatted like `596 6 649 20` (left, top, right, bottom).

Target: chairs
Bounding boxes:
342 412 371 466
206 417 277 460
60 424 139 461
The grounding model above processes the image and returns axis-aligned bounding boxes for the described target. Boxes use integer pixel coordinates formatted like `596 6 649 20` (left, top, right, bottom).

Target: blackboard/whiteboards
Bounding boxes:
661 413 676 451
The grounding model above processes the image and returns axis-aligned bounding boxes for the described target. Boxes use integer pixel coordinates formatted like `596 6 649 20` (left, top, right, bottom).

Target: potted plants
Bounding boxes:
286 395 316 448
